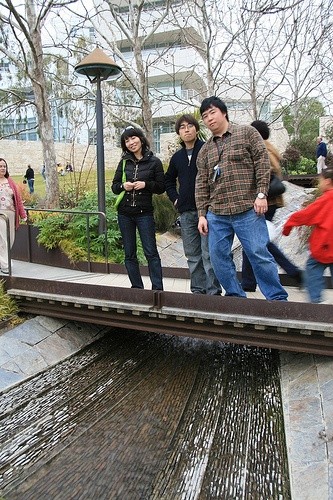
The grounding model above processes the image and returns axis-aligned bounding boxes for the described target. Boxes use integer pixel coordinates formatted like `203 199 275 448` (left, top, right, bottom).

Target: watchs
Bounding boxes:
257 193 267 200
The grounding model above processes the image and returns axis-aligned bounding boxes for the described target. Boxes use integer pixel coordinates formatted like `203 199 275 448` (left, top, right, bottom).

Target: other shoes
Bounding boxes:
1 268 9 273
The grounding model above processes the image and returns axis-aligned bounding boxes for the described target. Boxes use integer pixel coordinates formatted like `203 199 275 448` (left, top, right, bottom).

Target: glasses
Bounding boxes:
179 123 193 130
121 125 138 136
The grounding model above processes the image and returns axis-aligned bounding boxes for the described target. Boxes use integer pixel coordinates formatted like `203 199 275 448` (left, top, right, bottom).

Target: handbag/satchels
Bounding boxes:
23 176 27 184
267 174 285 199
114 159 127 211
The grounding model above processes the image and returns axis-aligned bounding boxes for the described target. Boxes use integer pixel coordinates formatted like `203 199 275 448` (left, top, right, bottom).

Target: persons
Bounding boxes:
22 164 35 193
56 162 64 174
283 167 333 303
316 136 328 174
66 161 73 174
193 96 289 303
0 159 29 279
164 113 224 298
238 120 305 292
111 128 165 290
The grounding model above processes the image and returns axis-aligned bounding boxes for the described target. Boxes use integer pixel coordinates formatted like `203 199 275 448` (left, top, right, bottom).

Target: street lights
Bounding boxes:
75 45 122 254
